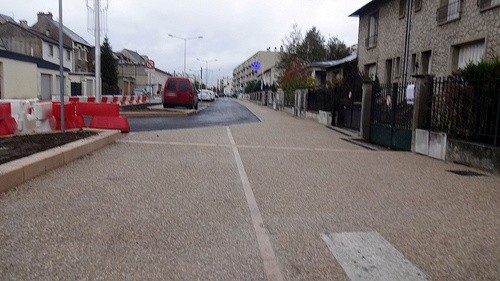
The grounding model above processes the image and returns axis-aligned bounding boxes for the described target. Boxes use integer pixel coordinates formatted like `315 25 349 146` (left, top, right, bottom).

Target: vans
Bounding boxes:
161 77 200 109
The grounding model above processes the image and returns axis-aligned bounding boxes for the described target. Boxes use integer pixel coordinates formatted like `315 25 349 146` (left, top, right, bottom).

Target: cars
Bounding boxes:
196 89 224 102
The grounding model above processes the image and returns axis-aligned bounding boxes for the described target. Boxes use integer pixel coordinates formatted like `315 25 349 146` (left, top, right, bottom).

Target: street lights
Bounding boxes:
168 34 204 77
197 58 219 89
